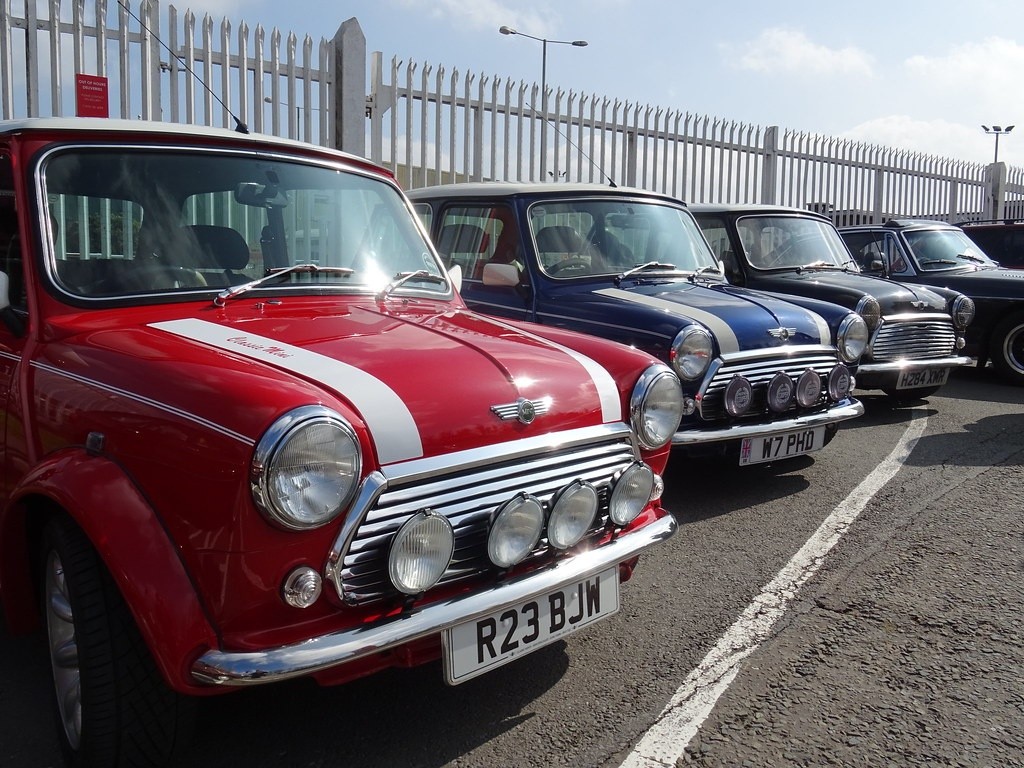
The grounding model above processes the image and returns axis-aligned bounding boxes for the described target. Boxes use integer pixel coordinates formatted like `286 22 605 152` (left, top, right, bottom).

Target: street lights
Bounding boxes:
498 25 588 181
264 96 328 143
981 124 1015 163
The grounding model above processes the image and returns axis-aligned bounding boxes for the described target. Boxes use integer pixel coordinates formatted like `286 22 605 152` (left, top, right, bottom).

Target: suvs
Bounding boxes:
949 217 1024 269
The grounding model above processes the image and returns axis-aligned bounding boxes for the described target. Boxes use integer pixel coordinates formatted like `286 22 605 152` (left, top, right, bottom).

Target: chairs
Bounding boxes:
50 210 103 296
536 223 589 274
436 223 490 279
863 251 888 272
140 222 266 287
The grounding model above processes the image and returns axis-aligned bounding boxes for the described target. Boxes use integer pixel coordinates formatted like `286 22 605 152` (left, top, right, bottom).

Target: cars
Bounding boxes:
579 203 1024 402
0 115 685 768
352 180 869 466
